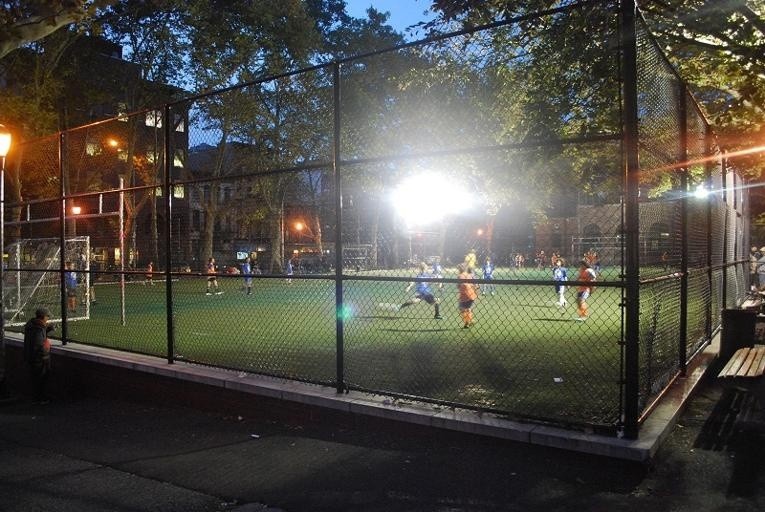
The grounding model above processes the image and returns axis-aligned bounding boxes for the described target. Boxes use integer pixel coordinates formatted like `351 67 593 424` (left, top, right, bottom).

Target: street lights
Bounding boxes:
0 124 12 321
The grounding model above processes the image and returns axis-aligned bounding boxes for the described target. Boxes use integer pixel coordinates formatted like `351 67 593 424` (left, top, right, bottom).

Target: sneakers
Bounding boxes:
205 291 225 296
393 298 592 329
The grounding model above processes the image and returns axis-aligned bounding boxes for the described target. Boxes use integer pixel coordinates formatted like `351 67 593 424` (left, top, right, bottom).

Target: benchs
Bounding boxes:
717 345 765 427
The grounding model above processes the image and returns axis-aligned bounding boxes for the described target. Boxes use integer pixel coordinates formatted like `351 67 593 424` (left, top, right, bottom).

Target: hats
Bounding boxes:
35 307 55 318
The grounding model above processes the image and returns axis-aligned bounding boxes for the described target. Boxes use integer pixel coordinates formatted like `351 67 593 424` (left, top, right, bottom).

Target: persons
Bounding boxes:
22 307 60 407
